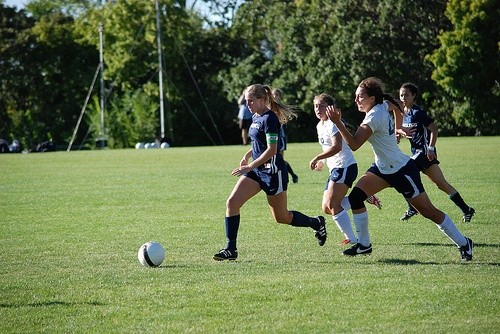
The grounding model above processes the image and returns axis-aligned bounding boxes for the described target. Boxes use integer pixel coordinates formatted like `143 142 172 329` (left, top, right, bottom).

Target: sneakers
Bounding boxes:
400 206 418 222
213 247 238 261
338 239 358 246
457 236 473 263
365 194 382 210
313 216 328 246
293 175 298 183
342 243 373 257
462 207 475 223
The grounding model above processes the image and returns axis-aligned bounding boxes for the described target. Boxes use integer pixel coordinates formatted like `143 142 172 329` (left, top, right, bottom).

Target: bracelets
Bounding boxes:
427 147 435 151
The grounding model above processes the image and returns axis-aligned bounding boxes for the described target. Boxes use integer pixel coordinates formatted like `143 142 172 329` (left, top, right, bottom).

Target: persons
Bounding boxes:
268 88 298 183
325 76 475 262
400 82 475 222
214 82 327 260
309 92 383 243
237 90 255 145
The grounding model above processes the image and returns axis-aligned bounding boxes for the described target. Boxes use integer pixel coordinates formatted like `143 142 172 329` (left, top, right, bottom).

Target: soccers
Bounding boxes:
138 241 165 267
